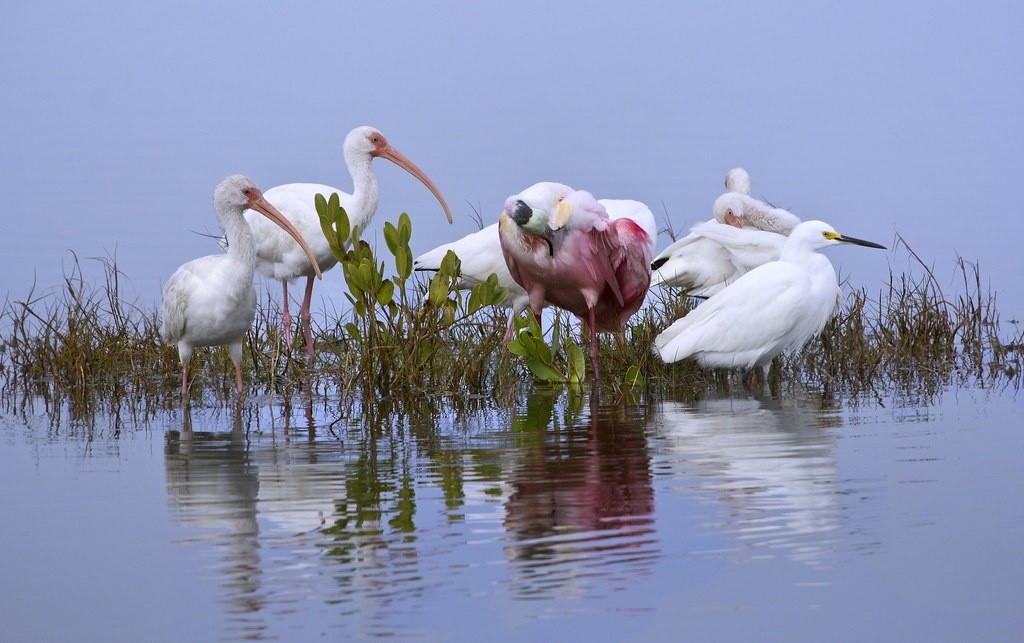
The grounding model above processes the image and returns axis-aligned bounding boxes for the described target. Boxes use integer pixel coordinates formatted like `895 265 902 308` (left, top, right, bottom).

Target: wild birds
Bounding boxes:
413 181 658 383
158 176 323 407
219 126 452 357
649 168 888 380
